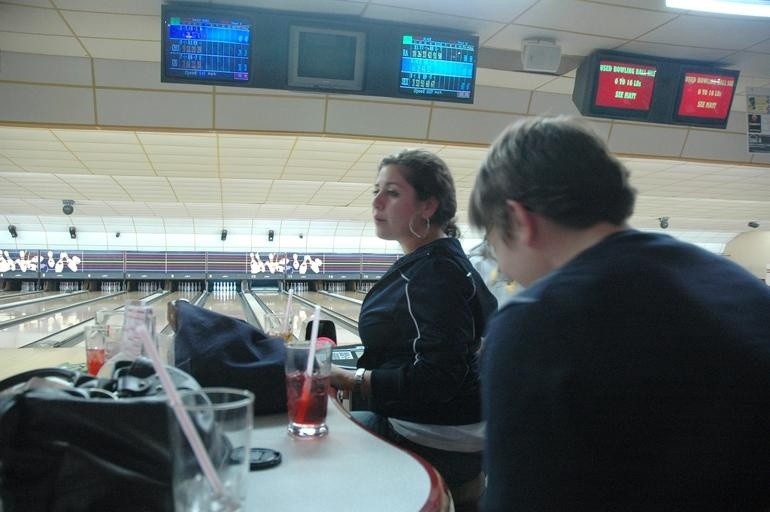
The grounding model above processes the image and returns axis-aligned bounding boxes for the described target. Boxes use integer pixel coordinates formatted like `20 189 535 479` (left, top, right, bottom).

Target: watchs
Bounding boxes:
354 368 365 396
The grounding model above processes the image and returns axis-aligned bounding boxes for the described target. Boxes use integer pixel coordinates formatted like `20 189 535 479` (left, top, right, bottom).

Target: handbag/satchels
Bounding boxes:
167 298 321 422
0 357 233 511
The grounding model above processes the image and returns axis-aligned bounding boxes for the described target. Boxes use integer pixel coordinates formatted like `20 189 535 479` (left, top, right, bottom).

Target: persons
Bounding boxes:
318 148 498 488
468 114 770 511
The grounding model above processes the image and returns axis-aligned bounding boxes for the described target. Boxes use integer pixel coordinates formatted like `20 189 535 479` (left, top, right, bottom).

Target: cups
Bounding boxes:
83 325 106 377
166 388 254 512
285 341 331 438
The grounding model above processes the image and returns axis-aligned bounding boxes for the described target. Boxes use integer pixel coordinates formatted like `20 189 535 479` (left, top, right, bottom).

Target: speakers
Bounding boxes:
69 227 76 239
221 231 226 240
8 225 17 237
268 231 274 241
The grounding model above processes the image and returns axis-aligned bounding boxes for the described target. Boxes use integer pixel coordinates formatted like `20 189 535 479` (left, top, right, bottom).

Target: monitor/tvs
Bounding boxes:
157 6 256 84
286 25 368 92
663 57 741 130
574 47 667 124
389 30 481 103
